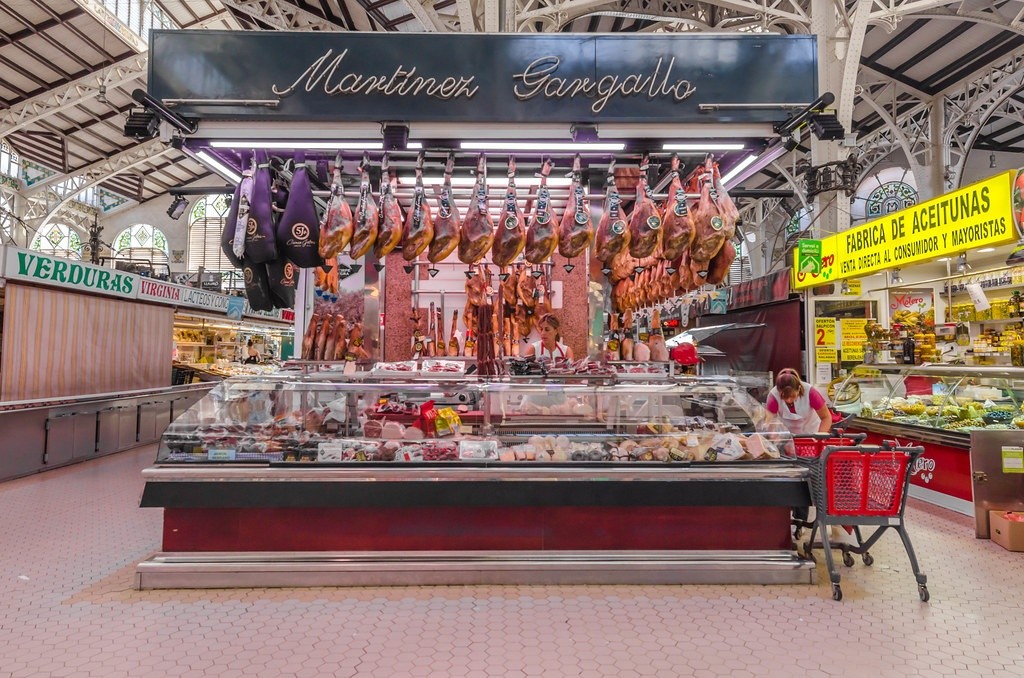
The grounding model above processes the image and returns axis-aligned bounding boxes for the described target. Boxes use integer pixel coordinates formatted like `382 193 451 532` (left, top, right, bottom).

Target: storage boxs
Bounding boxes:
989 510 1024 552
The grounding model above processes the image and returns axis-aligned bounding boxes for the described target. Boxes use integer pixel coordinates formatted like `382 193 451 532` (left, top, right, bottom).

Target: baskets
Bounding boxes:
826 451 910 517
785 438 855 459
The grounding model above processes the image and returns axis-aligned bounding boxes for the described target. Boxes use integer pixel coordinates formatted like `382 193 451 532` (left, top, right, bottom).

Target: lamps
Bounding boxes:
166 195 189 221
892 267 903 284
184 139 769 185
957 251 972 271
124 106 161 143
569 122 599 142
379 120 410 150
840 278 850 293
807 109 845 141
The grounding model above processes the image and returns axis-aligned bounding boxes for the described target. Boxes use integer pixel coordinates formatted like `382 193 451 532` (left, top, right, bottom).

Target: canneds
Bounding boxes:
863 317 941 365
972 329 1002 347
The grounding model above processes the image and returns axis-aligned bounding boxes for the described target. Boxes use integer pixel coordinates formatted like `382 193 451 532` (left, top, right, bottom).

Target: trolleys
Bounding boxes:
792 439 930 602
772 429 868 530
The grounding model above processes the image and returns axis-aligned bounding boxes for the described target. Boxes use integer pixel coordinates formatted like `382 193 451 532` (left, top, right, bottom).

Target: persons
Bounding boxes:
765 368 832 440
526 313 574 361
